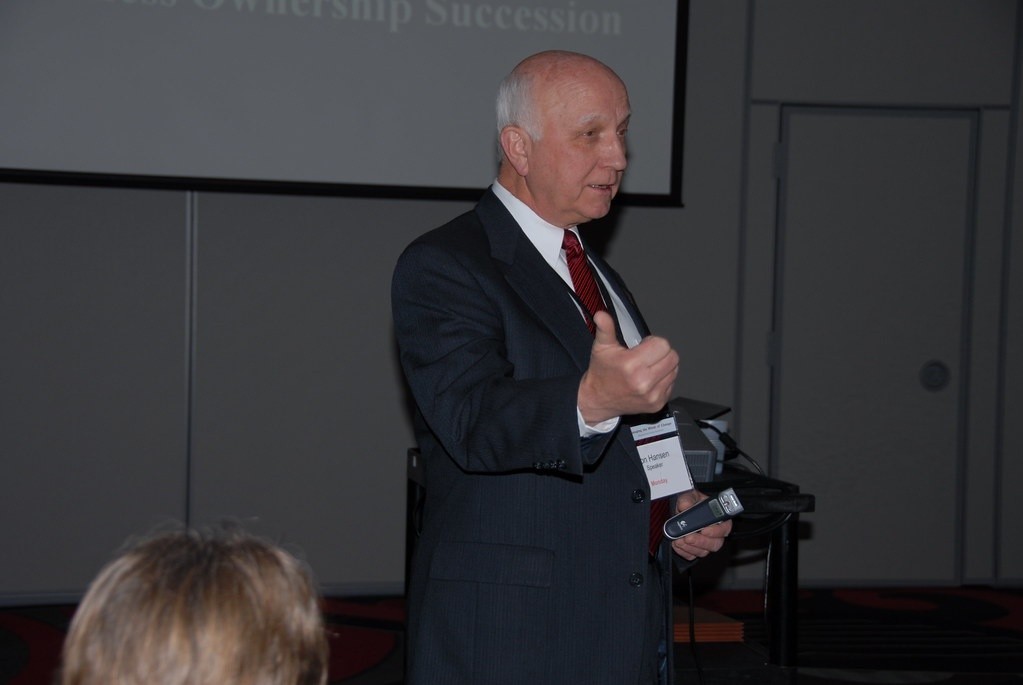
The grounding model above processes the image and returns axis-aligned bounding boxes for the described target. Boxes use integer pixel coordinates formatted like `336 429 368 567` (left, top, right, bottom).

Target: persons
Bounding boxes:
62 518 334 685
389 51 735 685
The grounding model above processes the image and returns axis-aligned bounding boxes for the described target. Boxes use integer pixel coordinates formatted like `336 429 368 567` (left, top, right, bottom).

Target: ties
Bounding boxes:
562 230 671 558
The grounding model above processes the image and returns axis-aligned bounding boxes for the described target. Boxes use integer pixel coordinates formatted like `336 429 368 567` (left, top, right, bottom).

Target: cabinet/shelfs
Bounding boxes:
403 449 817 684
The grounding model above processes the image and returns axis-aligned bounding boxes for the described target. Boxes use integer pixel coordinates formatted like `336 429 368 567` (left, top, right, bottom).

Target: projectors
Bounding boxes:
672 403 718 482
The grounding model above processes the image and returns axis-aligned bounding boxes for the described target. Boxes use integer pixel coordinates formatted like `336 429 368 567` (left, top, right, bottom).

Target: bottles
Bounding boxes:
699 420 729 476
